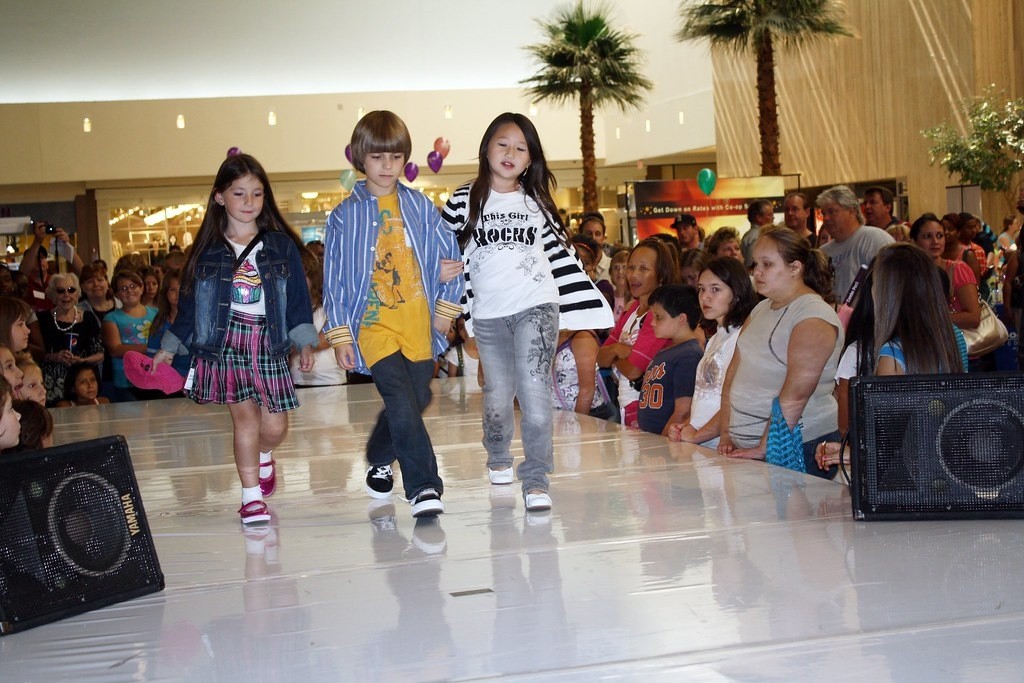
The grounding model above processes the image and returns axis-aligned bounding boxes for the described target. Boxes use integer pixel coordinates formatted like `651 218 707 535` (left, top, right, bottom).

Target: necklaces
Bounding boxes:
53 304 78 331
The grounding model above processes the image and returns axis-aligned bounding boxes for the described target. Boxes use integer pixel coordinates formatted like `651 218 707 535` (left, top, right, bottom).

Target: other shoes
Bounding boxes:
526 510 552 527
241 512 279 549
238 499 271 524
259 458 277 497
524 493 553 511
488 467 514 484
490 483 515 500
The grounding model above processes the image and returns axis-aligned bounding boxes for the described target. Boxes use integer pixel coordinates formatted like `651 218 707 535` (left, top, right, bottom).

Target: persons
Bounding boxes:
0 183 1024 485
320 109 464 517
151 155 321 526
438 111 616 513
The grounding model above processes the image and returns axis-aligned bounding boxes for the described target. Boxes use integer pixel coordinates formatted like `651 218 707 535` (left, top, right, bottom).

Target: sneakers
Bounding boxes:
397 488 444 518
364 465 394 499
401 515 448 563
368 499 397 535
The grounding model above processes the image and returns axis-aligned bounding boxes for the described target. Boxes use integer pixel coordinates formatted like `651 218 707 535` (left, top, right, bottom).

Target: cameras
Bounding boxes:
44 224 57 235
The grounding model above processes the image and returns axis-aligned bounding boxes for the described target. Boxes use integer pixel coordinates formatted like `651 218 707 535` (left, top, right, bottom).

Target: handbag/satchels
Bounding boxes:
607 403 622 426
963 249 993 308
630 313 648 391
764 398 808 473
949 260 1009 357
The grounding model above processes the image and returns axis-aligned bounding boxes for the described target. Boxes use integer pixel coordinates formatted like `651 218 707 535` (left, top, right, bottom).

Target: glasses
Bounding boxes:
55 286 78 294
115 283 139 293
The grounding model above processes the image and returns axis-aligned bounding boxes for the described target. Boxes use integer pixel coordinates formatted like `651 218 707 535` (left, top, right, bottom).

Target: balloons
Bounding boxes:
434 137 450 159
339 169 357 191
697 168 717 195
227 147 241 158
344 144 354 163
427 151 443 174
404 162 419 183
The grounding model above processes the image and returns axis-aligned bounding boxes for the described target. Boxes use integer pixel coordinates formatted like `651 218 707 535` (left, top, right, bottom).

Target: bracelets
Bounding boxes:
159 349 173 361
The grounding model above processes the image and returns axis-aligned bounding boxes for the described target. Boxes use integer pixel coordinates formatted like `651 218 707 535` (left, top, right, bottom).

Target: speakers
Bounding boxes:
848 371 1024 521
0 433 165 636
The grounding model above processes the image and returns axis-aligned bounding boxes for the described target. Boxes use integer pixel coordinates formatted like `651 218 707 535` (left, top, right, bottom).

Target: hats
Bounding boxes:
123 350 186 394
671 214 696 228
